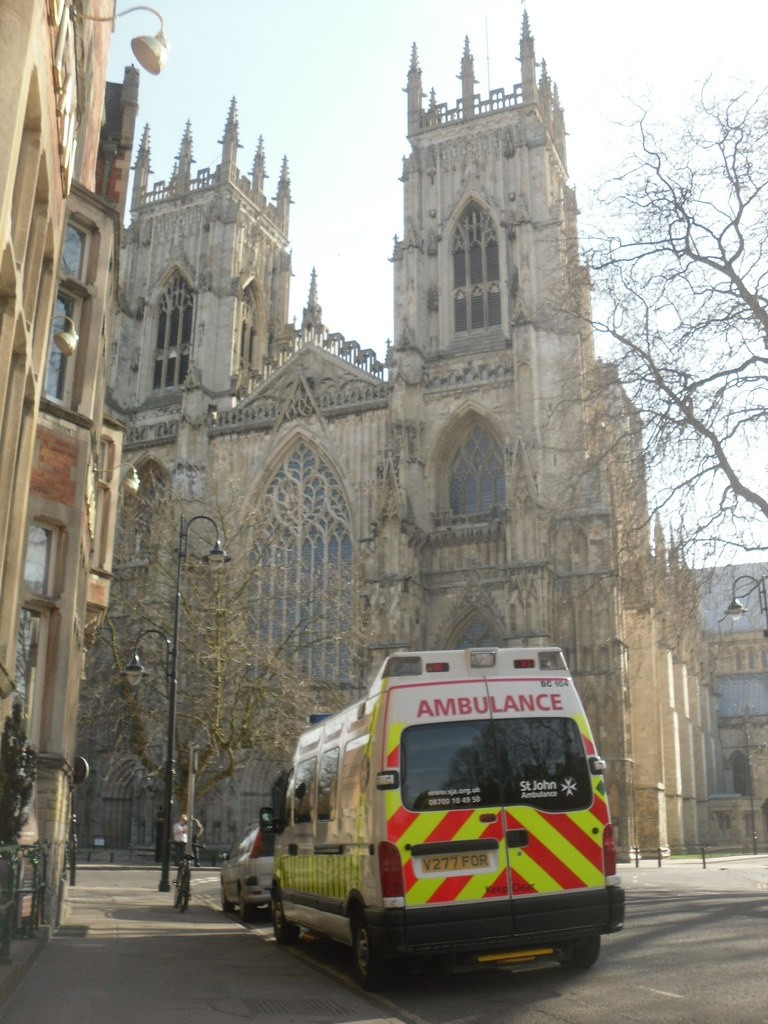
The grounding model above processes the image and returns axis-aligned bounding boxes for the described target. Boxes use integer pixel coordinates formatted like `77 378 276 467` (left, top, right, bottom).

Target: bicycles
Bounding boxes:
169 839 204 913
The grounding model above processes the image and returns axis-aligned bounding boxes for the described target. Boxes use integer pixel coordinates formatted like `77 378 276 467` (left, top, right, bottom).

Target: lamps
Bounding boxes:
93 462 140 495
65 2 170 77
50 315 80 356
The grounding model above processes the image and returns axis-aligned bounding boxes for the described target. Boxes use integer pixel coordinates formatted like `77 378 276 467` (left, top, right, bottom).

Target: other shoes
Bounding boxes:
195 863 201 867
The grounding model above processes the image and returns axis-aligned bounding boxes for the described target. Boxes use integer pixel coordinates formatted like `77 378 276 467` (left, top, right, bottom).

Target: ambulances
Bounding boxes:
257 647 628 991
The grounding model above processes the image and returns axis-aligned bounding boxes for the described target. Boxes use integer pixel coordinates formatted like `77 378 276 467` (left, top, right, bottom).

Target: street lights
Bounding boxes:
724 574 768 639
156 514 234 892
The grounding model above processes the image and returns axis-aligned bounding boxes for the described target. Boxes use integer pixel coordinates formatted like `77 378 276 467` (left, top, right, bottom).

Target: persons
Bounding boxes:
172 815 204 867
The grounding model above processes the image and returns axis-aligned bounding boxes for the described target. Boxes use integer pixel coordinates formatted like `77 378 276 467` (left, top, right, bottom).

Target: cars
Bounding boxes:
220 819 276 924
